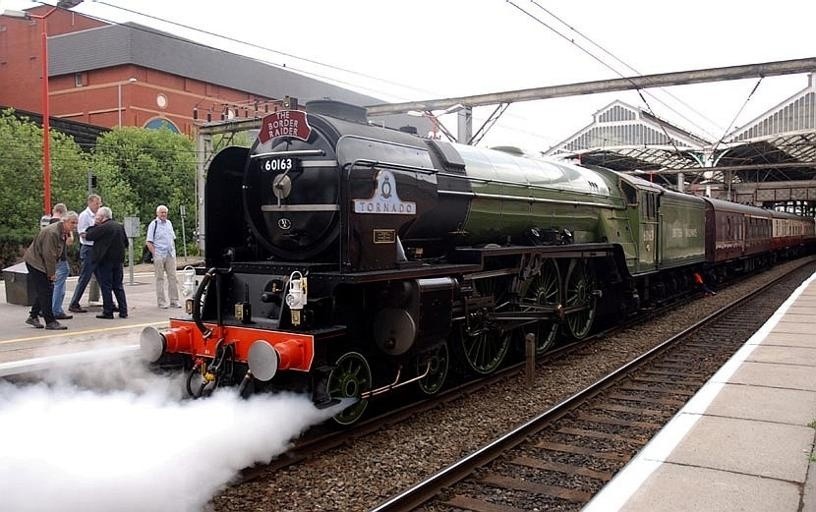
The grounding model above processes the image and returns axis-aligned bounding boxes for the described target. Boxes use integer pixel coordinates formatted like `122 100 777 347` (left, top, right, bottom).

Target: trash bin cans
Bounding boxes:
2 262 36 306
176 266 195 302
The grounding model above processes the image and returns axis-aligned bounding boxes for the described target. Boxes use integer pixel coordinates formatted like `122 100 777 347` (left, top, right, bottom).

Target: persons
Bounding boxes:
147 205 184 309
39 202 75 321
81 207 130 318
70 194 121 315
22 210 78 331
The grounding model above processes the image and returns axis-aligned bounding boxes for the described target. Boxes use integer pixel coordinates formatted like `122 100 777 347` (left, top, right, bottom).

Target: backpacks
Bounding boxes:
143 245 153 264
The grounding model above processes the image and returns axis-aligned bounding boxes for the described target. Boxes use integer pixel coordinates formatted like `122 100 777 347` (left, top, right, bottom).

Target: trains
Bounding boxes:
140 95 810 426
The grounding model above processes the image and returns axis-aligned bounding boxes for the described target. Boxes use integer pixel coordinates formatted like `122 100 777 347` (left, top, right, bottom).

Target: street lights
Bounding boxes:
1 1 80 216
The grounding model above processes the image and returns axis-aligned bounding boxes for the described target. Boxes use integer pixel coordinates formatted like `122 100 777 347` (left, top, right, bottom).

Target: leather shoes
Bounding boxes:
69 305 88 312
25 316 44 328
119 314 128 318
58 313 73 319
46 321 68 330
96 313 113 319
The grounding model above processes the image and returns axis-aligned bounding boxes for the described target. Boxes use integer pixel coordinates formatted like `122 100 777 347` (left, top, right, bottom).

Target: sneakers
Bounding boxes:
157 302 182 309
